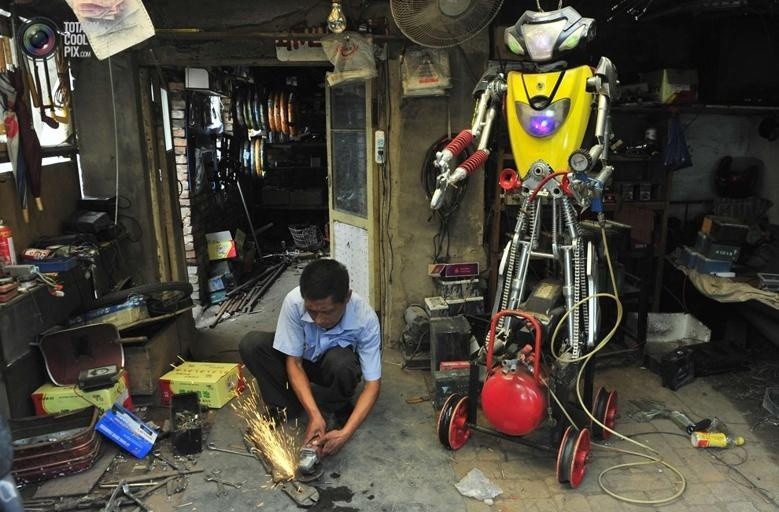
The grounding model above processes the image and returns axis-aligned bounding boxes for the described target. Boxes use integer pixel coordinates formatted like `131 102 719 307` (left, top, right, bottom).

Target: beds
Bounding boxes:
666 198 779 352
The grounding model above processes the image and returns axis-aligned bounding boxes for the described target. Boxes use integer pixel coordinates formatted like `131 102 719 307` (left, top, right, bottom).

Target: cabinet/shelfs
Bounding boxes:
260 144 328 239
488 104 670 364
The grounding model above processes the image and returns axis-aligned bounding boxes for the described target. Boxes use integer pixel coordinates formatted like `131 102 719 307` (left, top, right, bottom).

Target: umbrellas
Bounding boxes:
0 38 31 223
2 36 46 214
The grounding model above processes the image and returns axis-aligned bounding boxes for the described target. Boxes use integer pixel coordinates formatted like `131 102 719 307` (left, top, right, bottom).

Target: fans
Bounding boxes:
390 1 505 49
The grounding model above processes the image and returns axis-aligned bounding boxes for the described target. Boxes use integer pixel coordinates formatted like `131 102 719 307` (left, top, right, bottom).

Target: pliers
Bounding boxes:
405 396 431 403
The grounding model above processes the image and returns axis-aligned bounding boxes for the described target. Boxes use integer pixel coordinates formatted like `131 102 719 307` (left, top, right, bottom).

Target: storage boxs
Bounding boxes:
31 309 244 459
626 313 712 358
262 190 290 206
291 190 323 206
205 229 248 262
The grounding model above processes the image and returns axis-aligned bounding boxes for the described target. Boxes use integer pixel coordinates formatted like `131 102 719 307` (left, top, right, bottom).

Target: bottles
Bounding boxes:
691 430 744 449
0 220 18 266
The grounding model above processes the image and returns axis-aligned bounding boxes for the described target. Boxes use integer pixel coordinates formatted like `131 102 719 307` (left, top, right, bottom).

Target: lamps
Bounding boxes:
326 1 347 33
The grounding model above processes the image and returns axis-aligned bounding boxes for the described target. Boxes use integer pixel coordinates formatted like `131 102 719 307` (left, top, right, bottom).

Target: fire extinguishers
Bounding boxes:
0 218 18 279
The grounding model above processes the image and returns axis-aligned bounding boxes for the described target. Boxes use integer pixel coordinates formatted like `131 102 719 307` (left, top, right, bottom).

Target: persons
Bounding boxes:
239 258 383 456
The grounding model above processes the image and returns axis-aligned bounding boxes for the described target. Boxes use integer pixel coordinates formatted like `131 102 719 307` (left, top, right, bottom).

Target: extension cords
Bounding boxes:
375 130 385 164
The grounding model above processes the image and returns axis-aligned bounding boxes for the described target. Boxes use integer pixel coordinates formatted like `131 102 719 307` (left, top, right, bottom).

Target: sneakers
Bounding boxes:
259 403 300 429
335 404 355 428
